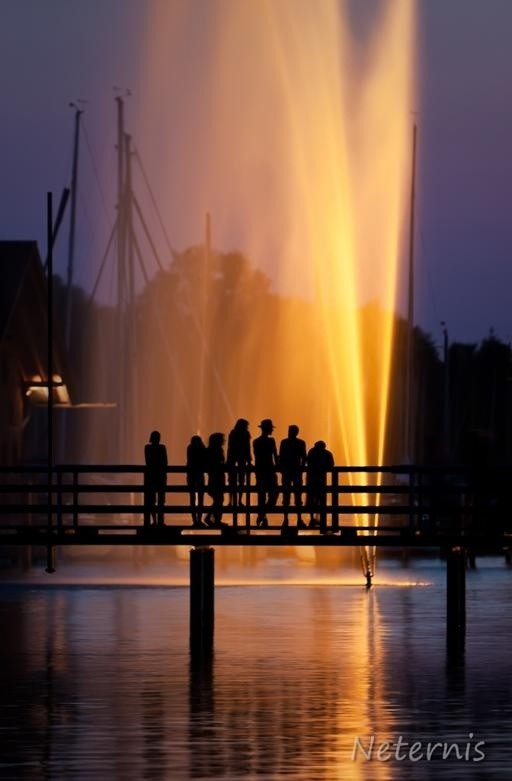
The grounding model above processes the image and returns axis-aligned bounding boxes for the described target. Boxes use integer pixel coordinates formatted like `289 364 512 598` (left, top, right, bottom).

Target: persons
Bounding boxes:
204 434 227 526
144 431 167 526
254 419 280 529
279 425 308 528
305 441 335 528
184 435 206 524
226 418 252 507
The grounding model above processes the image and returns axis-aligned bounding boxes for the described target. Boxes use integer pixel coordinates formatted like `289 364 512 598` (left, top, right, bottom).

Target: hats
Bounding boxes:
256 420 276 428
